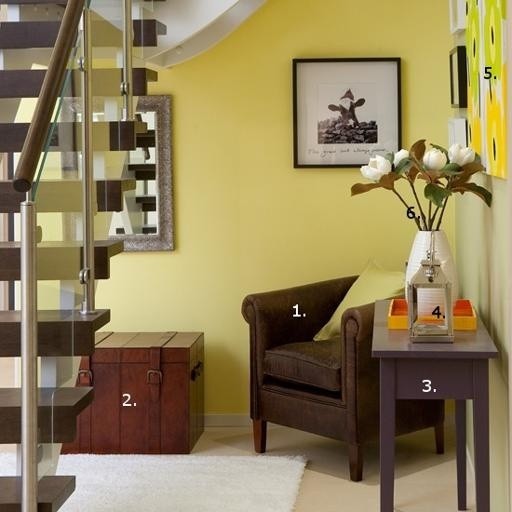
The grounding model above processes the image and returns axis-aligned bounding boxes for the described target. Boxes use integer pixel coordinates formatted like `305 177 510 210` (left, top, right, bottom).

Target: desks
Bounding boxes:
370 298 499 512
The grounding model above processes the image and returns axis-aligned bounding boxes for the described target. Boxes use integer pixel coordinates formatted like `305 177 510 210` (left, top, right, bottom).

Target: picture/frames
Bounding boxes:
290 56 403 170
448 1 469 154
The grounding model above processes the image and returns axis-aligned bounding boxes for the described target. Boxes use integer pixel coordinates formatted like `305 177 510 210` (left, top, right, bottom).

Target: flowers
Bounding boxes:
348 139 492 231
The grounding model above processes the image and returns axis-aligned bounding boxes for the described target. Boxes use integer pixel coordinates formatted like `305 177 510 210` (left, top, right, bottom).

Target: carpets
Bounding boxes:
2 453 310 510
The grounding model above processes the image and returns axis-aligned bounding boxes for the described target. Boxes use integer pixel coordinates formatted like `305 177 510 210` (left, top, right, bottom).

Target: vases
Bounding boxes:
405 230 457 309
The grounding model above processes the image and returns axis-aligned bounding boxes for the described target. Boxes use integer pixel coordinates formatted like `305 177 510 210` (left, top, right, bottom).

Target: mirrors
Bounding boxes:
108 93 175 253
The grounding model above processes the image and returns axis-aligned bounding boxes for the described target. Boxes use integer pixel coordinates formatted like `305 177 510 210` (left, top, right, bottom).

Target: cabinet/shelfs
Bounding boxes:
56 331 206 455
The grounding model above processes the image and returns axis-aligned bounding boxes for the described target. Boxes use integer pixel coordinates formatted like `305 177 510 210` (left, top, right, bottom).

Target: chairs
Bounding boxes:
241 276 446 480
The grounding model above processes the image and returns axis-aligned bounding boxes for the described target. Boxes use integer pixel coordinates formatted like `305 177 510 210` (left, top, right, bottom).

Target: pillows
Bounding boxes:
315 265 405 342
